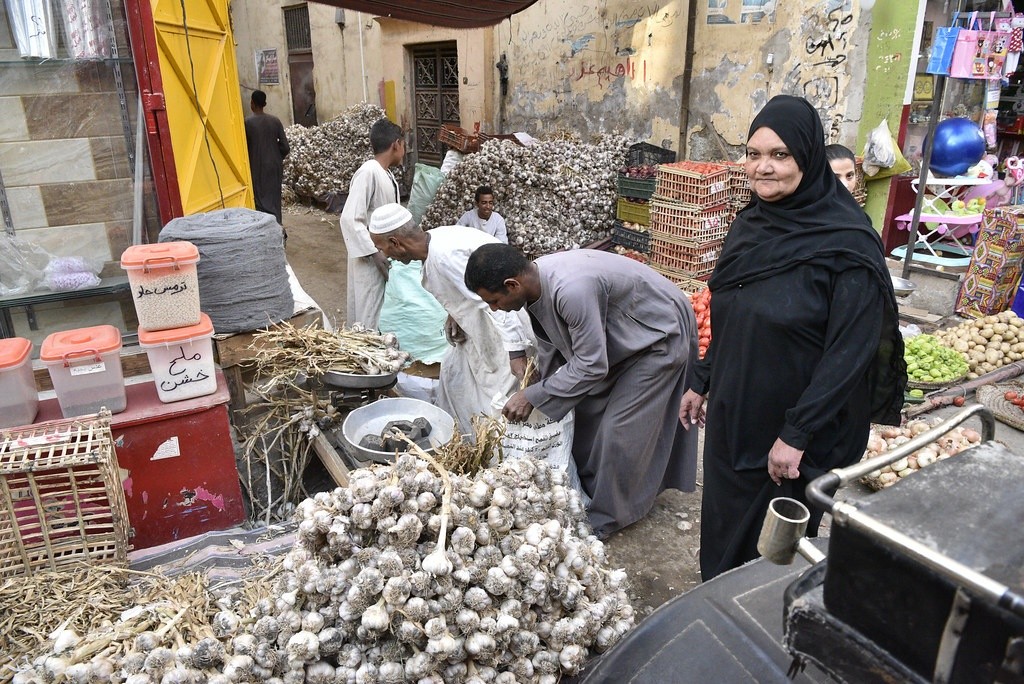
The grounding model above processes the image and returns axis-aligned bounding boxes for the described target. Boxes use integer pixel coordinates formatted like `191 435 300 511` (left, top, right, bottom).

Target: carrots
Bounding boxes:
1004 392 1024 412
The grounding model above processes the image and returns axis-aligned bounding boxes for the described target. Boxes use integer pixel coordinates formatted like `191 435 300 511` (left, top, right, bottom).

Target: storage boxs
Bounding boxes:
0 241 219 434
610 141 754 297
439 124 520 153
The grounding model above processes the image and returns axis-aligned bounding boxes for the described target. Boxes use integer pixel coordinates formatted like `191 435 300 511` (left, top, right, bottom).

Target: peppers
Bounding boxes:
901 335 967 398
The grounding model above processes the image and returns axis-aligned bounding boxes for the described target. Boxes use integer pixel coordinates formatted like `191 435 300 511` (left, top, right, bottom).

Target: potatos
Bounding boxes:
932 310 1024 378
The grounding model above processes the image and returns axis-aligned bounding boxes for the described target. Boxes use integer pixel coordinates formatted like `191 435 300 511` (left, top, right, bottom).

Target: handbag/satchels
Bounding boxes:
861 120 913 181
972 11 1012 75
1003 1 1022 52
491 391 575 472
926 12 978 75
862 119 896 178
950 11 1002 80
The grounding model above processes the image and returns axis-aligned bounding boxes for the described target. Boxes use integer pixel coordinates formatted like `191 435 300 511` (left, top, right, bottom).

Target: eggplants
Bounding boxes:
631 163 657 179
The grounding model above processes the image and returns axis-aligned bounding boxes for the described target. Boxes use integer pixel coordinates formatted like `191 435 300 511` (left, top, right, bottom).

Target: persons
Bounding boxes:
456 186 508 244
244 90 290 240
366 205 539 441
465 243 700 539
340 120 405 330
827 144 856 193
679 95 908 582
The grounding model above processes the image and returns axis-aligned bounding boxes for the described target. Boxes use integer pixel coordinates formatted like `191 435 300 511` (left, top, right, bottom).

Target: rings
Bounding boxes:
782 472 787 475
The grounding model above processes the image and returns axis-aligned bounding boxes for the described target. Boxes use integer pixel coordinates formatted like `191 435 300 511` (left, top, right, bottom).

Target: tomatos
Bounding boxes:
654 160 749 357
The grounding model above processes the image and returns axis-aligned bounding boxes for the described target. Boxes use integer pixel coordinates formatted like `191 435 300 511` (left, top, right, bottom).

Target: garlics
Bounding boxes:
0 102 636 684
863 419 982 489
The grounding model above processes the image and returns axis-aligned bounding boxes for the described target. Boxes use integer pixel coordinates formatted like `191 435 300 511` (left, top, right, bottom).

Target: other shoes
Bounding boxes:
593 530 617 543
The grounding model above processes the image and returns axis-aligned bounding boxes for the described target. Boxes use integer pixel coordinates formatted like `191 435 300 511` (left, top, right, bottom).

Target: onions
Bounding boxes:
953 396 964 407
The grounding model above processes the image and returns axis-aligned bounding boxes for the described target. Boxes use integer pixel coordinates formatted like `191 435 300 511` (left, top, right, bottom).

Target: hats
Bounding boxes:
369 203 412 234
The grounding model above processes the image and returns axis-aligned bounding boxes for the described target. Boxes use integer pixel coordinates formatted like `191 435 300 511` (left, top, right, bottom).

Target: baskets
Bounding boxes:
905 375 967 394
976 379 1024 431
